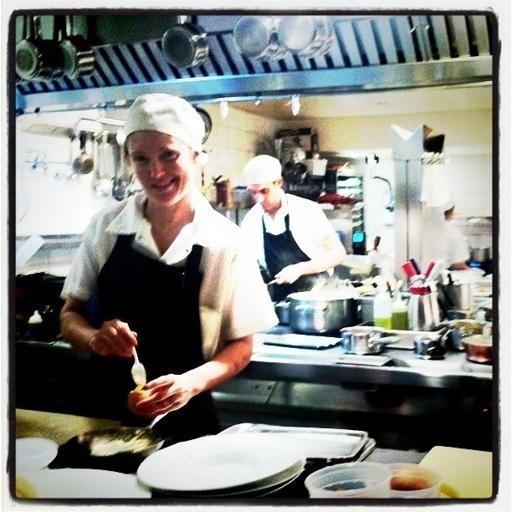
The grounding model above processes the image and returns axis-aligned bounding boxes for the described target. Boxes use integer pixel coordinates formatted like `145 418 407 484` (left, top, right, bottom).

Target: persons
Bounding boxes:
57 94 280 430
238 153 349 297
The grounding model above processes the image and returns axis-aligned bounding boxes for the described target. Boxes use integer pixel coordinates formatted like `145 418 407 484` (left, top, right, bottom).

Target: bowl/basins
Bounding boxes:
386 462 445 500
460 336 495 363
302 461 393 498
288 291 355 335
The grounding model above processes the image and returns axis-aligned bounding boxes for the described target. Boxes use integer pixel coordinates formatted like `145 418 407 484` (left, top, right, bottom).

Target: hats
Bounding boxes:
114 92 206 156
241 155 282 185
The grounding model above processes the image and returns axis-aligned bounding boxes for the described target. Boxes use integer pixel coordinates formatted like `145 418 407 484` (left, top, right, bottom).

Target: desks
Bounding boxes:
16 315 509 400
9 406 505 505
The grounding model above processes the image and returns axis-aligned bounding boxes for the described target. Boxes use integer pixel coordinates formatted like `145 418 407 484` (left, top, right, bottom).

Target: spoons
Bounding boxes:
111 135 128 200
72 125 96 175
124 321 149 389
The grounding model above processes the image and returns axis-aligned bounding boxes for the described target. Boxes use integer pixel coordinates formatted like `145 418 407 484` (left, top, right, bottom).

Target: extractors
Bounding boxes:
17 14 494 117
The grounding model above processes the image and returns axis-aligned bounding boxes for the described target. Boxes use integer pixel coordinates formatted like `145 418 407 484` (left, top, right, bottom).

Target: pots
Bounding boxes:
342 330 401 355
59 410 167 468
469 247 490 263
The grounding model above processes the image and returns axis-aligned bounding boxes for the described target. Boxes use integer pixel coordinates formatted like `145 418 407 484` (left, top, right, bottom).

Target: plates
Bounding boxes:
16 468 152 499
137 435 306 502
217 424 377 467
15 436 60 477
